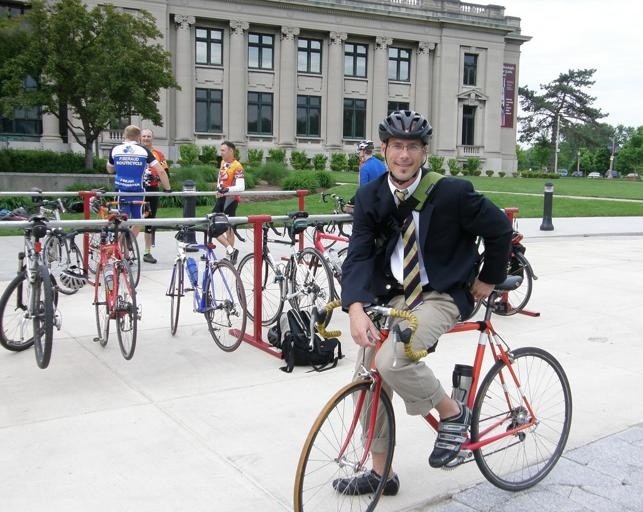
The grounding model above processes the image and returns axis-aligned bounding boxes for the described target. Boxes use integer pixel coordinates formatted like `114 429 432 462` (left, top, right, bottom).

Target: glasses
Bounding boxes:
386 141 425 154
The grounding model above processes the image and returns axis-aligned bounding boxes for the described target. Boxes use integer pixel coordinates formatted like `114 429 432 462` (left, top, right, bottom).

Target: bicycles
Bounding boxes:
331 247 374 312
474 232 538 317
293 295 574 510
165 213 246 353
0 197 73 369
233 211 334 329
320 192 352 249
31 187 83 280
85 197 140 289
91 199 151 361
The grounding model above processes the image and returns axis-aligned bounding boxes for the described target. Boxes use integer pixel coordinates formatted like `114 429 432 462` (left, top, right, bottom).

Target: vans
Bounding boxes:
541 167 549 173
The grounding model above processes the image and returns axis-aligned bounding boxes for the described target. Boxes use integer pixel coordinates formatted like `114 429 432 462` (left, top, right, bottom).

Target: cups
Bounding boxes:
451 363 473 403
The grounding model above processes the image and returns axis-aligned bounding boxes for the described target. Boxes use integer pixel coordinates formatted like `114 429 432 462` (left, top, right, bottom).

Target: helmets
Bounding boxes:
59 270 87 289
358 140 374 150
379 110 432 144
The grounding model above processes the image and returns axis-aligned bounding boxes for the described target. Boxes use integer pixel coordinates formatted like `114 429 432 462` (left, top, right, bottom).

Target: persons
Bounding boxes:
106 125 170 265
343 140 385 217
206 140 245 268
332 109 514 497
133 128 170 264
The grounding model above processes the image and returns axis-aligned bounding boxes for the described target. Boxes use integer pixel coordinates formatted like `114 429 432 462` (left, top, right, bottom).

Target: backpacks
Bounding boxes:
278 308 342 372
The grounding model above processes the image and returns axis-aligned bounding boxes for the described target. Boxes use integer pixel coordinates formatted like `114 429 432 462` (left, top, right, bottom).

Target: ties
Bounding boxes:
394 189 424 311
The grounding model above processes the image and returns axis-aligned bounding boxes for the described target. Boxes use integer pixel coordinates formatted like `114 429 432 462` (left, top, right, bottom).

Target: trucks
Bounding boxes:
571 171 582 177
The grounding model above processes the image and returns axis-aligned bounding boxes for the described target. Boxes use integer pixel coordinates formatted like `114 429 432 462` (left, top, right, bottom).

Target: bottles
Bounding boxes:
102 261 117 290
185 257 197 285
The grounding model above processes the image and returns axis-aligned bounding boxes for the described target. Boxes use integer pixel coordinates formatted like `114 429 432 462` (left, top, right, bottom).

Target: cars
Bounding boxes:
561 169 568 176
623 173 639 181
557 168 561 176
605 170 618 178
586 172 600 179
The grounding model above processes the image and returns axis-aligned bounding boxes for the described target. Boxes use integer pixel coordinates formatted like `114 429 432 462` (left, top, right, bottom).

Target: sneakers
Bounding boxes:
333 470 399 495
429 400 472 468
144 253 156 263
230 249 239 265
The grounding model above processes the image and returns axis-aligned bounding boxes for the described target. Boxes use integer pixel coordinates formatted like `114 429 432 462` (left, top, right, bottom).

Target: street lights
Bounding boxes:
576 151 581 171
608 137 616 170
554 113 560 173
